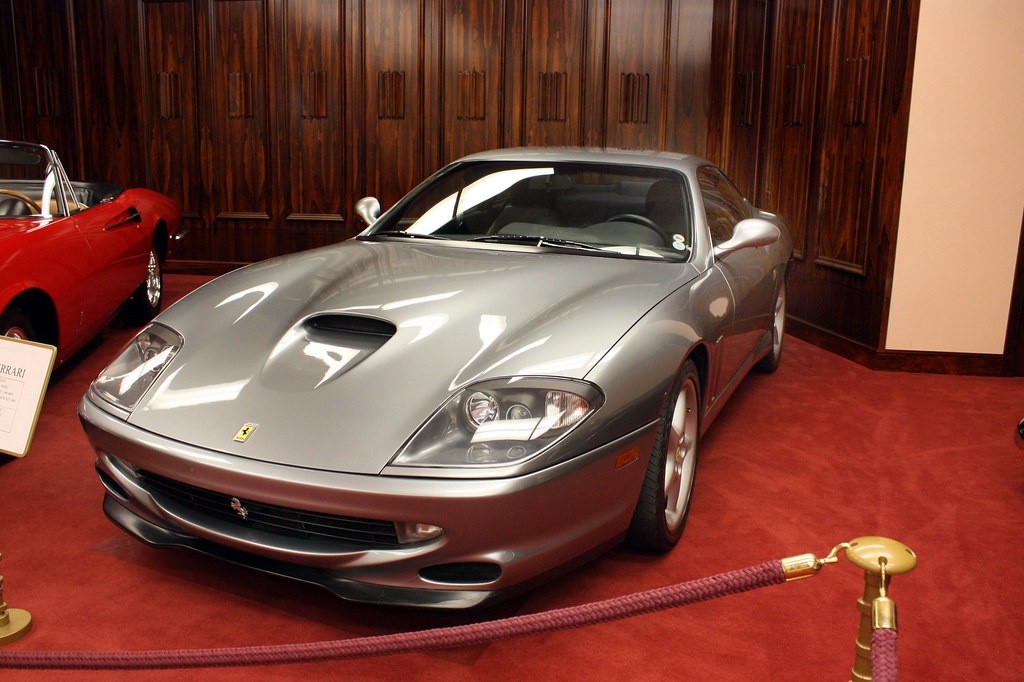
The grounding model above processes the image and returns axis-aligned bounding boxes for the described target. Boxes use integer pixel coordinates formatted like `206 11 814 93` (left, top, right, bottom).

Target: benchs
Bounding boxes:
0 179 94 217
551 192 647 227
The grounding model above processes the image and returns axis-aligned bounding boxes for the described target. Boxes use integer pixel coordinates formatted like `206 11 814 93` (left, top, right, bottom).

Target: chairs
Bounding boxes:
488 189 556 239
643 180 686 239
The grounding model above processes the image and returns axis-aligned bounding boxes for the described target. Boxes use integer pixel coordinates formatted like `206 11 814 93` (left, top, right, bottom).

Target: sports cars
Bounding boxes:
77 147 795 621
0 140 185 393
